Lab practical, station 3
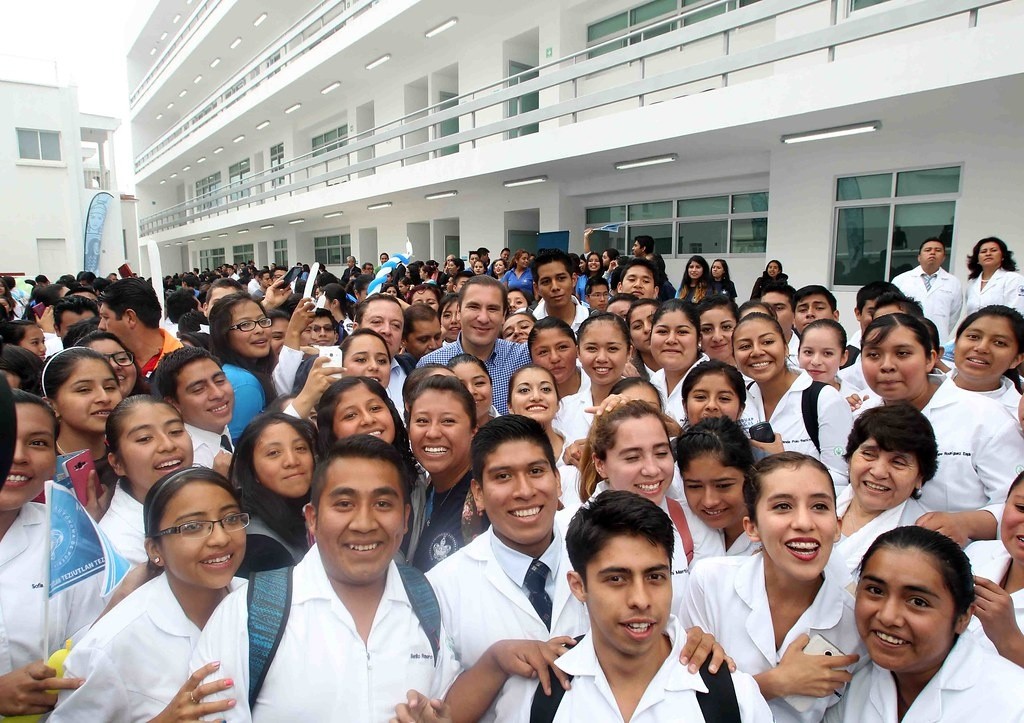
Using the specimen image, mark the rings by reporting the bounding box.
[191,692,197,703]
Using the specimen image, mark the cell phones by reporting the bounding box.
[276,266,303,289]
[319,346,343,379]
[32,302,45,319]
[749,422,775,443]
[63,449,103,506]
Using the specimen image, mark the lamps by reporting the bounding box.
[324,212,343,218]
[164,229,249,247]
[368,54,391,71]
[149,0,301,184]
[426,191,459,199]
[781,119,882,144]
[427,17,458,37]
[321,81,341,95]
[289,219,304,224]
[368,202,393,209]
[261,224,275,229]
[614,153,678,170]
[503,174,547,188]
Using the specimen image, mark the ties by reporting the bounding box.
[922,273,937,291]
[220,434,232,453]
[523,560,553,634]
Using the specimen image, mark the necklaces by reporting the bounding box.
[56,441,65,454]
[982,280,989,282]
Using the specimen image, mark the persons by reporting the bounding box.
[0,228,1024,723]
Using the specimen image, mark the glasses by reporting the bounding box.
[229,318,272,332]
[103,351,135,366]
[314,323,337,333]
[151,511,252,539]
